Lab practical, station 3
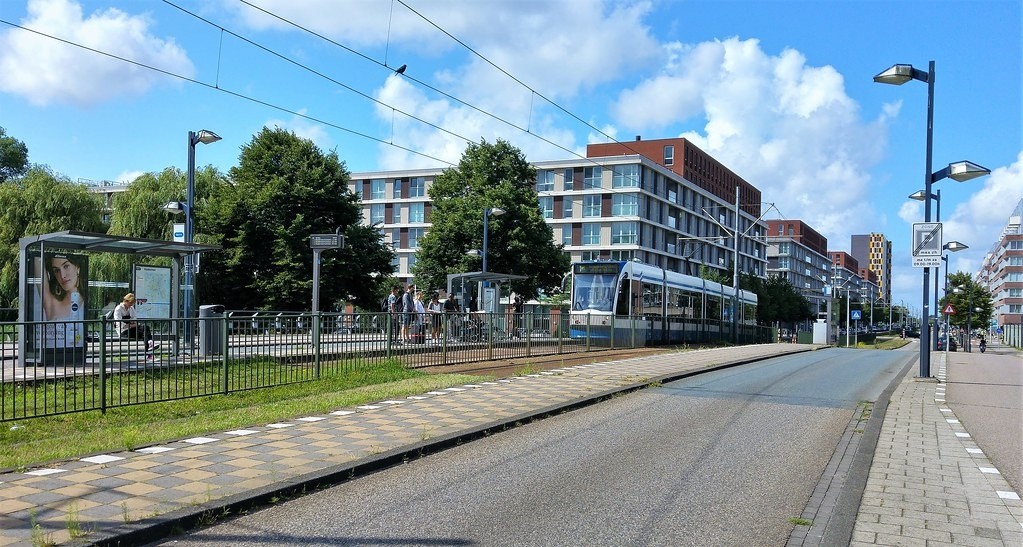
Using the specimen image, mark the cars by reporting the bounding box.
[838,324,921,339]
[938,331,957,351]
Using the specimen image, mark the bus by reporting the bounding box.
[561,242,757,347]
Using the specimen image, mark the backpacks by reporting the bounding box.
[396,292,409,312]
[381,294,389,311]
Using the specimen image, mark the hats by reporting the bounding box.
[124,293,134,303]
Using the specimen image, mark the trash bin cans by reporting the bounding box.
[199,305,224,355]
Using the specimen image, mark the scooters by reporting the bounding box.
[979,341,989,353]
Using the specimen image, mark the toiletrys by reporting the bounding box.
[69,293,79,330]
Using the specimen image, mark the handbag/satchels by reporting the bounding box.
[100,310,114,325]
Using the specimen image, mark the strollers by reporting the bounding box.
[453,315,487,343]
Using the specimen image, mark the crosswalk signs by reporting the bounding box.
[851,310,861,320]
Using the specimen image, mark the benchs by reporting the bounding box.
[87,334,176,355]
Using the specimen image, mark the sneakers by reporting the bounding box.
[148,342,159,350]
[146,354,160,362]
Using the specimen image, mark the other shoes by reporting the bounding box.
[401,339,414,345]
[391,340,403,346]
[433,338,440,343]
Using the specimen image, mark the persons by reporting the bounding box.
[414,291,429,345]
[427,293,443,344]
[979,337,986,346]
[453,299,463,337]
[469,294,478,320]
[512,297,523,326]
[442,293,458,344]
[388,285,403,346]
[402,284,418,344]
[34,256,84,347]
[113,293,161,362]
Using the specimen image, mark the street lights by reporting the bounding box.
[908,189,971,351]
[465,207,507,272]
[163,129,223,343]
[940,254,964,351]
[872,60,993,378]
